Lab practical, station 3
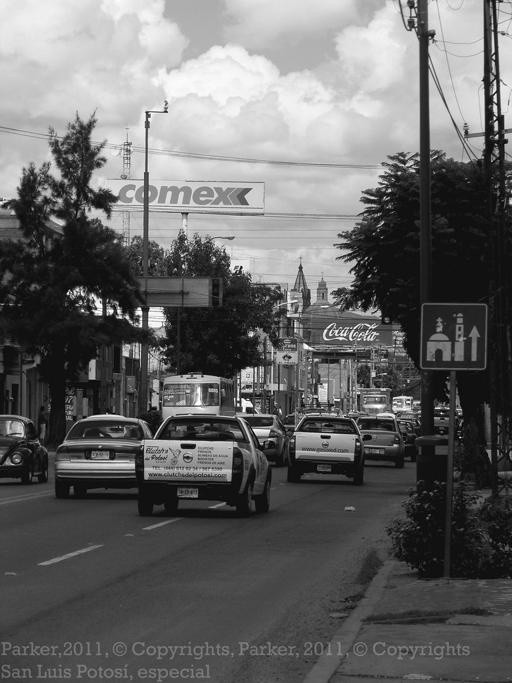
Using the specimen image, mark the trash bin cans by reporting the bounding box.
[415,434,454,485]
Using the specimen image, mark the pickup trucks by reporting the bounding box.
[285,412,372,485]
[135,412,272,517]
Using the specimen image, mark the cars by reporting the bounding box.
[54,411,159,500]
[282,387,464,462]
[355,415,406,468]
[231,412,291,466]
[1,414,49,484]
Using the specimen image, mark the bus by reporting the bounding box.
[159,371,235,425]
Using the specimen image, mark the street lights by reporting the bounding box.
[293,304,330,386]
[369,372,387,387]
[253,298,298,413]
[177,235,235,376]
